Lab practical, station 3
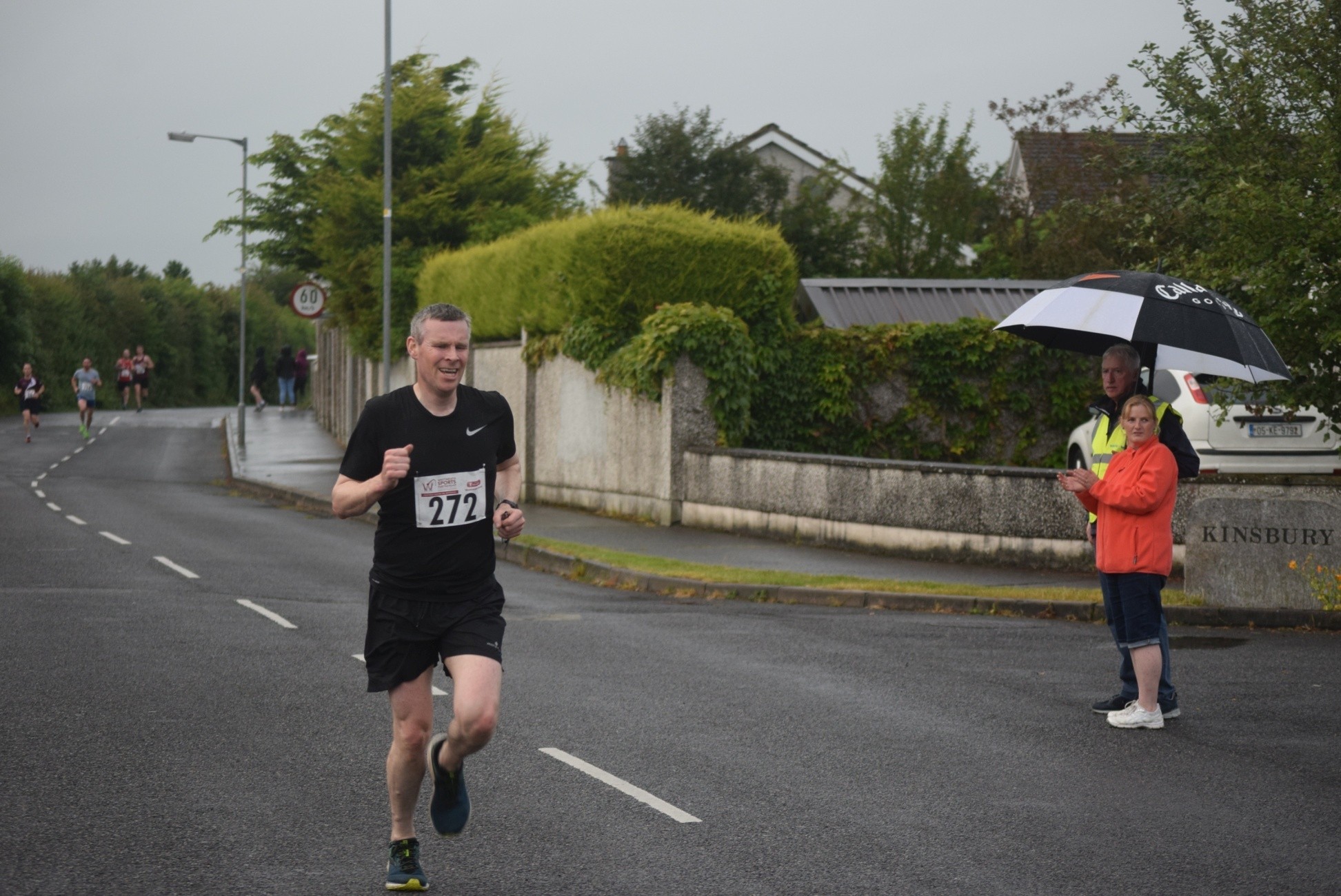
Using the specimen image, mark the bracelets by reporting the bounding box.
[494,499,518,510]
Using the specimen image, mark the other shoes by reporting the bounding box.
[79,423,89,440]
[24,434,31,442]
[254,400,267,413]
[34,422,39,429]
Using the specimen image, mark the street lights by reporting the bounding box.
[168,130,248,451]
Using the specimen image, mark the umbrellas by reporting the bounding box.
[993,267,1291,388]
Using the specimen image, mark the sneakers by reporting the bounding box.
[385,837,430,891]
[1092,694,1140,714]
[1157,692,1181,719]
[1106,700,1165,730]
[427,732,472,839]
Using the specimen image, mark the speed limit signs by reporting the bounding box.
[291,283,326,319]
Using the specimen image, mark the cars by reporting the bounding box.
[1141,364,1341,476]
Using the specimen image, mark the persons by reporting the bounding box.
[13,364,45,441]
[132,346,153,412]
[72,359,102,438]
[115,349,134,409]
[1084,342,1200,718]
[276,344,295,406]
[331,301,526,887]
[1056,395,1178,731]
[292,349,310,405]
[249,347,268,411]
